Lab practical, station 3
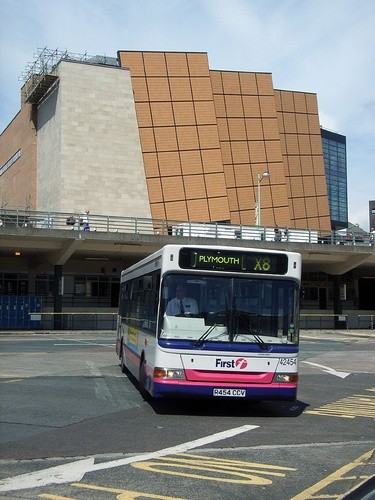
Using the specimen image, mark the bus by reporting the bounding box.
[115,244,305,407]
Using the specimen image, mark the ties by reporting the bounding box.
[179,300,185,315]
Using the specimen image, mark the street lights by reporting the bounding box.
[256,172,270,227]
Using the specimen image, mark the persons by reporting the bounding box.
[163,285,198,317]
[82,211,89,231]
